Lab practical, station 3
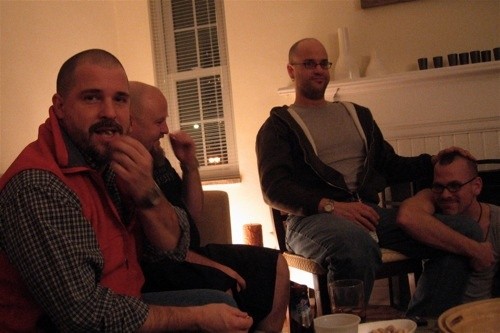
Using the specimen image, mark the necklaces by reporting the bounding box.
[477,201,483,223]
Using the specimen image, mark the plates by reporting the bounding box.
[437,298,500,333]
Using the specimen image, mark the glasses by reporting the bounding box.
[431,178,474,193]
[292,62,332,69]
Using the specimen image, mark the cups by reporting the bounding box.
[481,50,492,62]
[470,51,480,64]
[448,54,458,66]
[328,279,366,322]
[493,47,500,61]
[433,56,443,68]
[459,53,469,65]
[314,313,361,333]
[418,58,427,70]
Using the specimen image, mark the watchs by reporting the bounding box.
[323,199,335,213]
[136,181,163,209]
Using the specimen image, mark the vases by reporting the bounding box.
[334,27,360,81]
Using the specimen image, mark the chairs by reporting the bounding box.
[272,207,423,317]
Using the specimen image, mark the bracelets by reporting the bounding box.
[179,159,199,172]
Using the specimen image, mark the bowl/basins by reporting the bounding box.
[358,318,417,333]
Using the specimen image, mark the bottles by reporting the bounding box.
[352,194,379,243]
[334,28,359,80]
[288,284,316,333]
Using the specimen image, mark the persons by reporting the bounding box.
[255,38,500,333]
[0,49,291,333]
